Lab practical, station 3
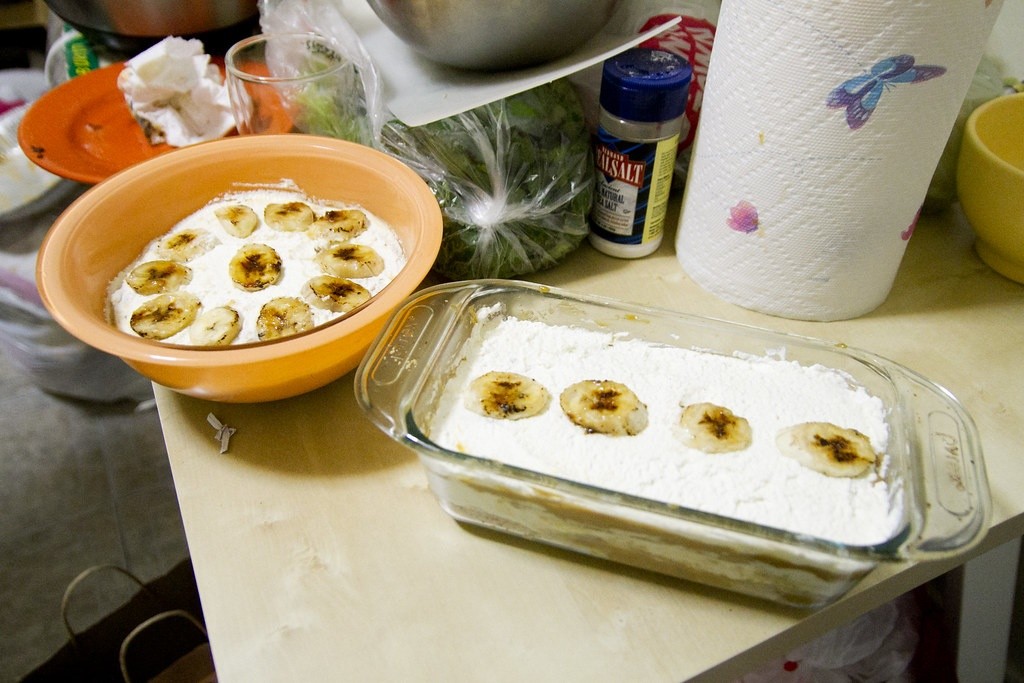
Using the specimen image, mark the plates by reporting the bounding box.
[16,59,296,184]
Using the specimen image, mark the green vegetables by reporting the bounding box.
[292,32,596,280]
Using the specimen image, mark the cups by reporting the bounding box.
[226,32,364,143]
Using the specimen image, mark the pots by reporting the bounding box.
[44,0,271,63]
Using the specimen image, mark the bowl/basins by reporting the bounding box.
[352,278,995,617]
[0,102,83,225]
[369,1,624,76]
[34,135,443,405]
[954,91,1024,289]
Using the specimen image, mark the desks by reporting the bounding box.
[150,198,1023,683]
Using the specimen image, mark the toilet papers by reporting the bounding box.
[670,1,1006,328]
